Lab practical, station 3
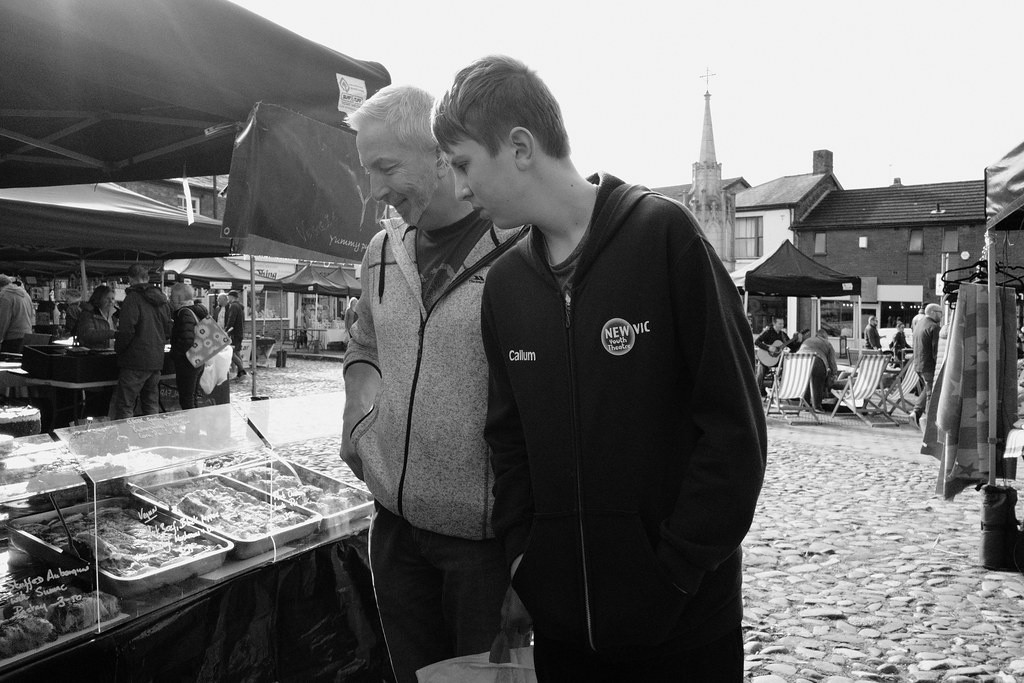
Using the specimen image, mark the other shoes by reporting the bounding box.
[815,409,825,414]
[909,412,920,430]
[235,370,246,382]
[763,395,767,402]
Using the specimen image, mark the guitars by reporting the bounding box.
[757,328,810,367]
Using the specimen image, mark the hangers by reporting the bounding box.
[941,255,1024,309]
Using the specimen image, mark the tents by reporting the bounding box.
[0,1,391,401]
[0,183,362,350]
[729,237,862,359]
[983,142,1024,573]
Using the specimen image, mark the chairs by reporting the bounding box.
[871,355,920,418]
[829,350,900,429]
[766,352,820,427]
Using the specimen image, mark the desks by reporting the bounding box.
[0,513,397,683]
[281,328,327,351]
[306,327,346,350]
[242,337,276,368]
[5,370,178,428]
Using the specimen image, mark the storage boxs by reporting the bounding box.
[20,345,177,384]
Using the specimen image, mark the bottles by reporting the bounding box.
[53,305,60,324]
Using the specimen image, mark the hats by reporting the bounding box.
[228,290,239,298]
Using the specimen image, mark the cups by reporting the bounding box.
[109,338,116,349]
[43,286,50,301]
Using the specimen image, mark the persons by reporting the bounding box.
[340,55,767,683]
[168,282,205,410]
[212,290,247,381]
[795,330,838,414]
[345,297,357,339]
[78,285,120,349]
[296,303,310,348]
[865,316,887,350]
[0,274,32,353]
[908,301,942,430]
[889,321,911,361]
[64,288,81,337]
[754,314,802,402]
[114,263,171,420]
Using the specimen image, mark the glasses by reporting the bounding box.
[934,310,943,314]
[874,320,878,322]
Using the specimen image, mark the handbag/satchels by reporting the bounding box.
[177,308,232,369]
[415,625,539,683]
